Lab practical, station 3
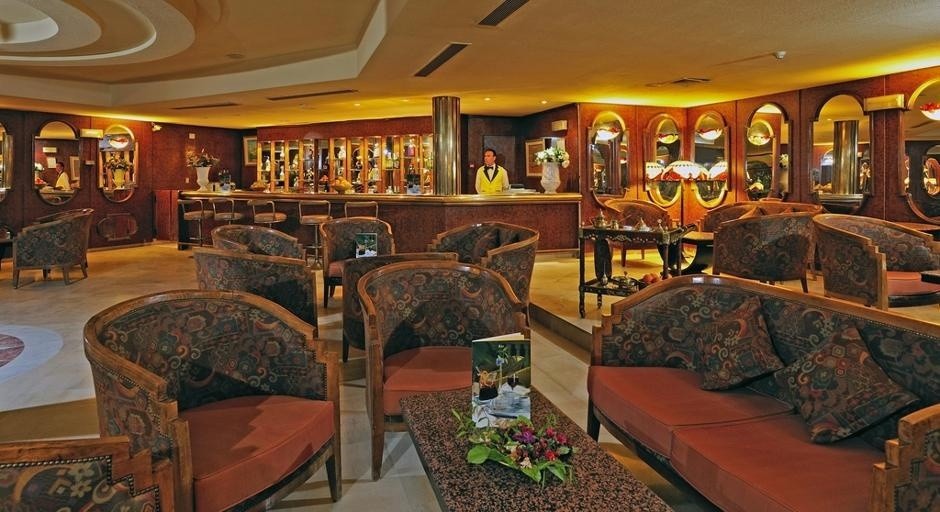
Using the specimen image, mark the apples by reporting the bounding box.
[642,272,672,284]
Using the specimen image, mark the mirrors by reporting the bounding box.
[96,122,140,204]
[33,134,80,196]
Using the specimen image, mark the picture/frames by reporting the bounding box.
[525,139,546,178]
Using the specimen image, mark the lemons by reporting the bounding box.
[332,176,351,186]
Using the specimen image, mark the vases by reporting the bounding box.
[111,168,126,189]
[196,166,211,191]
[539,162,561,196]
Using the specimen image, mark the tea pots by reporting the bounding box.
[590,208,609,228]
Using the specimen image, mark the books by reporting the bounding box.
[469,331,533,429]
[354,232,380,258]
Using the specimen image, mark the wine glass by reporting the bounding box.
[476,378,500,415]
[507,369,519,397]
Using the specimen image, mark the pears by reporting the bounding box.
[250,181,263,189]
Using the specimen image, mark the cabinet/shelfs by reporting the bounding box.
[254,132,432,194]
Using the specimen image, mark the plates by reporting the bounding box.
[512,406,530,413]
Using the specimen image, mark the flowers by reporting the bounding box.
[183,148,220,169]
[534,145,570,169]
[103,153,134,172]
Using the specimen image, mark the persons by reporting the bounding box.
[474,148,510,195]
[53,161,72,190]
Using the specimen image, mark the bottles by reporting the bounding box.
[653,219,665,232]
[264,154,272,169]
[279,164,285,179]
[338,146,346,159]
[385,152,400,169]
[386,184,393,193]
[322,180,331,193]
[406,138,414,156]
[279,144,285,160]
[322,157,330,170]
[220,167,232,194]
[612,219,619,229]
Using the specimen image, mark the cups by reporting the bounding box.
[516,395,530,408]
[488,399,509,414]
[358,242,373,256]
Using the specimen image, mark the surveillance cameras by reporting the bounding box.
[154,125,163,130]
